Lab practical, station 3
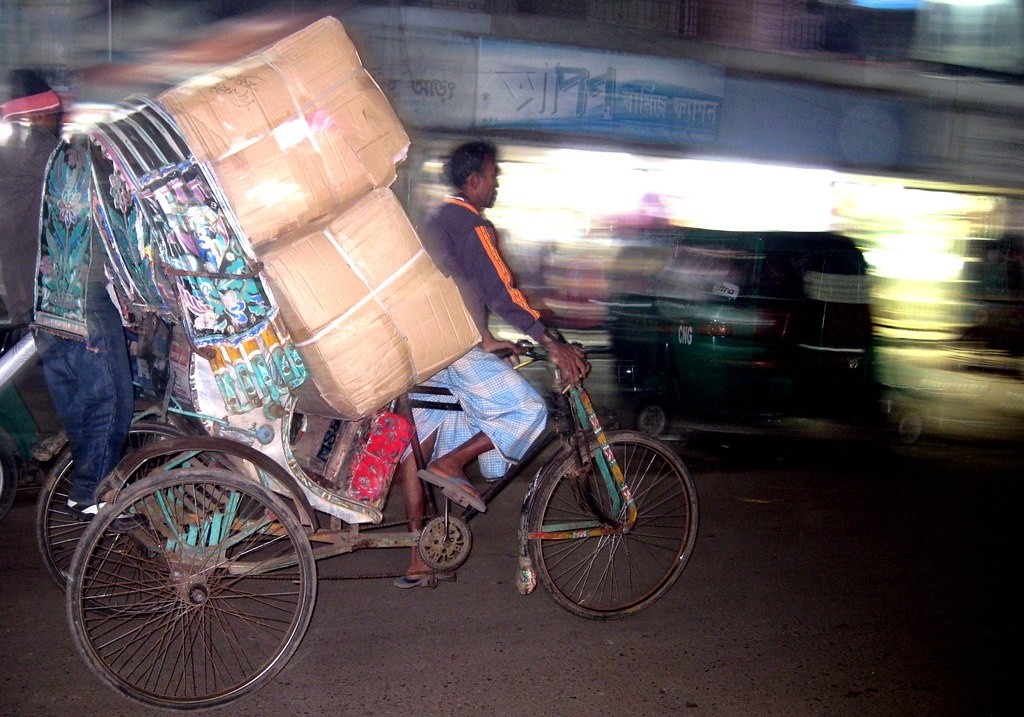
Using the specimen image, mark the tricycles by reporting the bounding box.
[32,308,702,713]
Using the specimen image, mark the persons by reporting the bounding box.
[0,68,142,535]
[389,139,590,587]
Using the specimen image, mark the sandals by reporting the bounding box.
[65,496,149,535]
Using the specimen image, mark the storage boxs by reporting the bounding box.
[77,8,410,246]
[254,185,482,423]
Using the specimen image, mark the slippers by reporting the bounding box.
[393,570,457,588]
[416,468,487,512]
